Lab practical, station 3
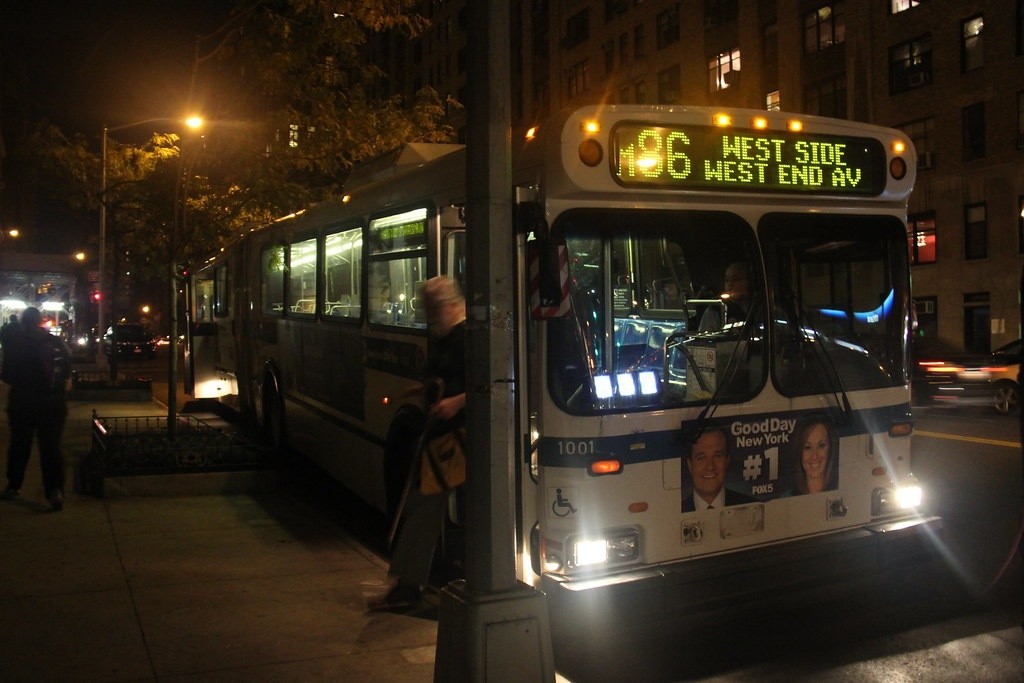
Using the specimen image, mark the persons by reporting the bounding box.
[697,260,794,346]
[366,273,482,613]
[684,416,839,514]
[0,306,71,510]
[0,313,74,373]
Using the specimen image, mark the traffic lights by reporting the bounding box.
[182,263,190,277]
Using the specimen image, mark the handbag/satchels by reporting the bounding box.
[381,402,431,490]
[41,333,81,403]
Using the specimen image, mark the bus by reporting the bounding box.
[188,101,945,623]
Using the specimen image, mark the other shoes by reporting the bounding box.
[46,490,66,509]
[0,486,22,500]
[367,584,418,608]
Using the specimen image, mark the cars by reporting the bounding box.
[88,318,157,363]
[980,339,1024,416]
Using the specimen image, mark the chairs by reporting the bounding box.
[612,318,689,370]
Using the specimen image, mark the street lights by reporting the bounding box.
[94,111,208,373]
